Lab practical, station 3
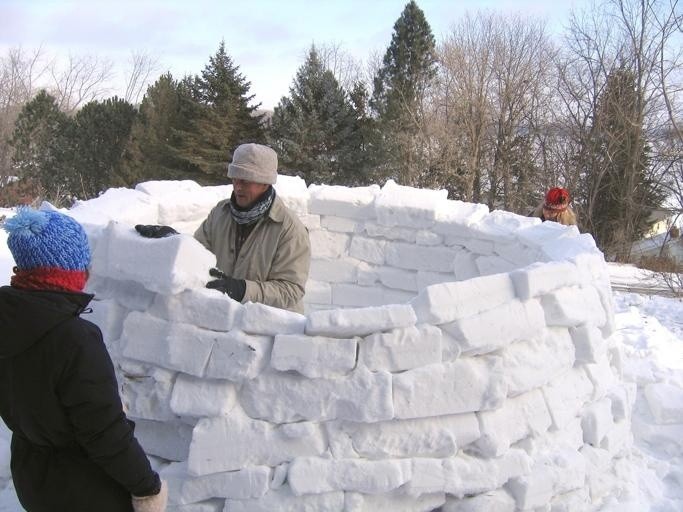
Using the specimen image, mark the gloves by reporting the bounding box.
[134,224,178,238]
[207,264,245,301]
[131,474,169,512]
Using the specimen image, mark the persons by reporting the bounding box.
[526,186,576,229]
[0,203,170,511]
[133,141,312,313]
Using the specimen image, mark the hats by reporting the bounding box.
[226,142,278,187]
[543,187,570,212]
[2,205,92,274]
